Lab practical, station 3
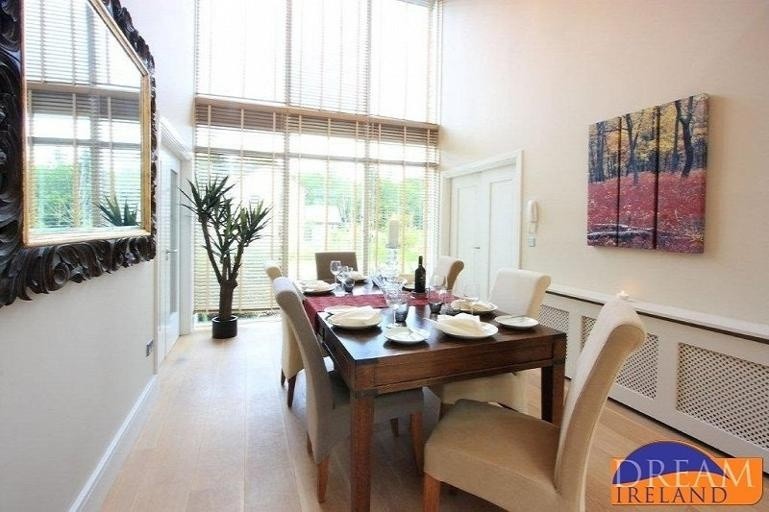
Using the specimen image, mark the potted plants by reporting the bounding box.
[176,172,276,340]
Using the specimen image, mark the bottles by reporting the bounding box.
[414,256,427,294]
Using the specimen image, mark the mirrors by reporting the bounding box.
[1,1,159,309]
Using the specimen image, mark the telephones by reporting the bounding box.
[528,200,537,222]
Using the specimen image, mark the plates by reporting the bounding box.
[324,298,539,346]
[299,277,336,296]
[403,282,431,290]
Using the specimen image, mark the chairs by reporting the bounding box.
[263,249,646,512]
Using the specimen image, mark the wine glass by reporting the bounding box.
[328,259,356,294]
[363,267,411,329]
[427,272,476,315]
